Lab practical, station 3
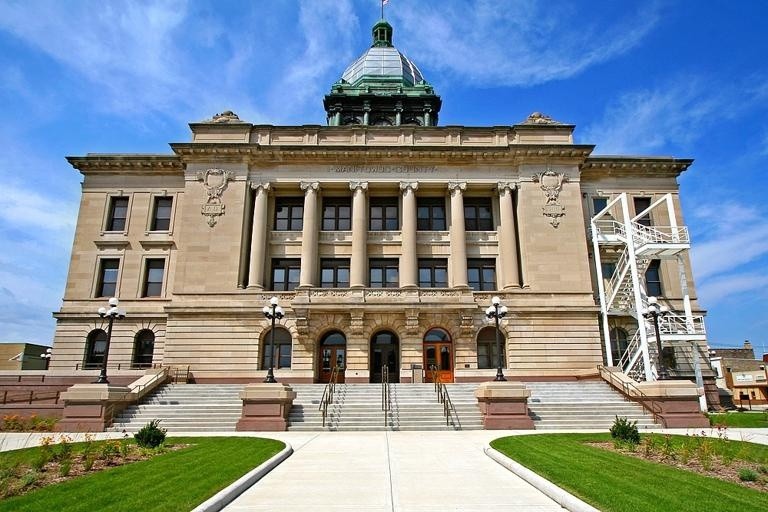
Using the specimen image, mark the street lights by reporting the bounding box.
[261,296,283,383]
[89,297,128,385]
[38,349,51,370]
[483,295,508,381]
